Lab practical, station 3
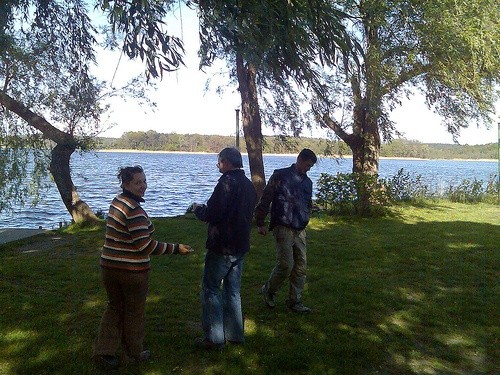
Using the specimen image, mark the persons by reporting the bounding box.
[193,146,257,351]
[94,164,195,375]
[255,148,317,312]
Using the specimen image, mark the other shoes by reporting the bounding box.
[197,337,244,350]
[129,343,156,364]
[285,298,310,311]
[261,285,276,308]
[96,354,122,371]
